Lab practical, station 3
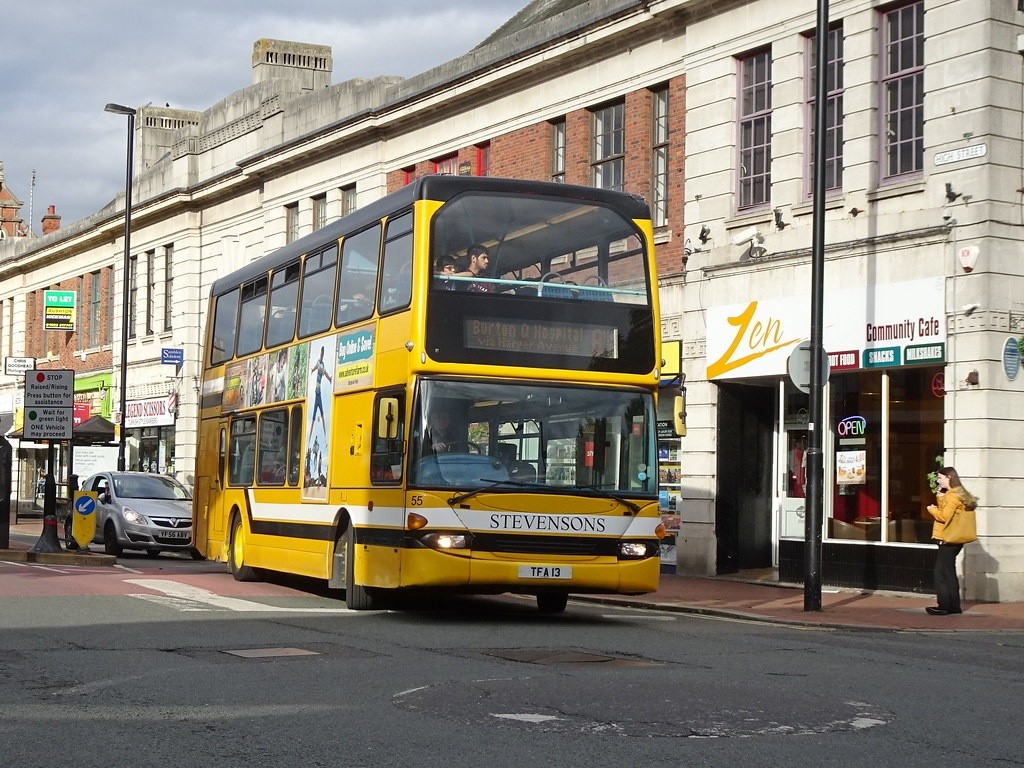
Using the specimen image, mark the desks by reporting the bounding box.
[854,520,880,530]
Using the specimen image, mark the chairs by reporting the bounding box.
[223,295,333,360]
[828,515,938,544]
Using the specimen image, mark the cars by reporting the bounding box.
[63,471,208,562]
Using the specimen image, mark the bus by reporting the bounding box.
[186,173,690,612]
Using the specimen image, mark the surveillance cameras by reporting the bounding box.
[732,227,758,246]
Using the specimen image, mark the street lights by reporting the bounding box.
[104,103,137,471]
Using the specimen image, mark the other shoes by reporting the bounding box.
[926,607,962,614]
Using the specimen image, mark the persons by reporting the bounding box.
[926,467,978,615]
[98,482,112,502]
[338,276,371,324]
[434,244,517,296]
[421,408,468,457]
[249,346,332,485]
